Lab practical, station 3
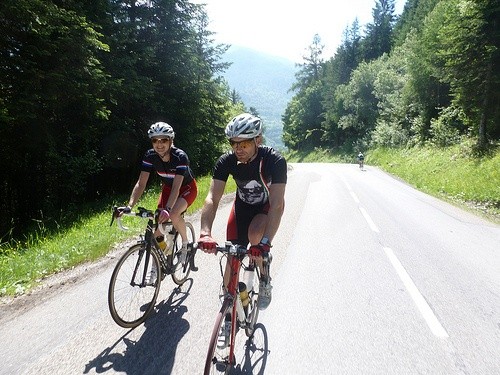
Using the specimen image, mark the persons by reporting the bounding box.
[198,113,288,349]
[357,152,364,167]
[116,122,198,284]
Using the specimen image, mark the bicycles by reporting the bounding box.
[359,159,363,169]
[195,240,272,373]
[108,206,198,328]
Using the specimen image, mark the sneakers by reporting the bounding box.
[256,283,272,310]
[217,326,231,349]
[145,270,157,284]
[181,245,188,264]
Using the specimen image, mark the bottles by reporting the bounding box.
[165,232,174,256]
[243,264,255,299]
[156,236,167,252]
[239,282,249,308]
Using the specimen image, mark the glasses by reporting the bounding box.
[230,138,255,149]
[150,138,169,144]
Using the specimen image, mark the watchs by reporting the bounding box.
[260,238,271,247]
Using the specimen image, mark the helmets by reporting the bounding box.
[225,113,262,138]
[148,121,174,139]
